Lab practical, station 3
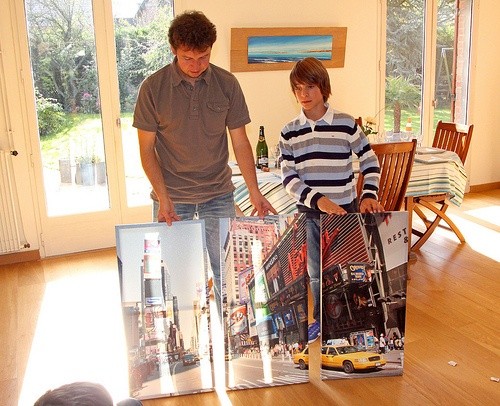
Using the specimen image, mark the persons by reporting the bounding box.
[244,332,399,354]
[34,381,143,406]
[281,57,386,345]
[132,10,281,323]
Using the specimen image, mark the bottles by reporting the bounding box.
[256,126,268,168]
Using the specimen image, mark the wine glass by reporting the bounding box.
[271,144,281,171]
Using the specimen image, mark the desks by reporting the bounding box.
[228,146,469,261]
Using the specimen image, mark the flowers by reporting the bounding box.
[361,106,388,137]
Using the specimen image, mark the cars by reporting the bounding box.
[293,347,309,370]
[183,354,196,366]
[321,344,386,374]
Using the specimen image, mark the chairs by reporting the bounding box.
[356,139,417,212]
[410,120,474,253]
